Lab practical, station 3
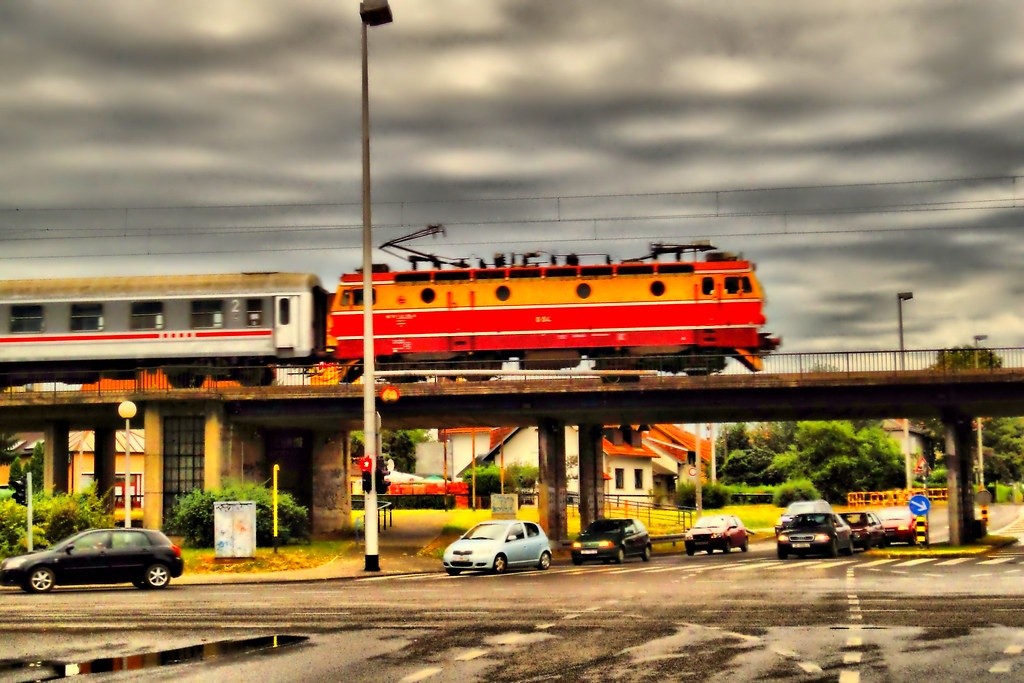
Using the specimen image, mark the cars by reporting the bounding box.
[0,527,185,594]
[837,512,887,550]
[441,519,554,576]
[774,512,855,559]
[570,518,652,565]
[877,505,917,547]
[684,514,749,557]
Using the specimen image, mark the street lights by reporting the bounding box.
[897,291,914,490]
[118,400,137,544]
[974,334,988,533]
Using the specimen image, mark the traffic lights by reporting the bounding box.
[359,457,373,494]
[375,454,392,494]
[9,478,27,503]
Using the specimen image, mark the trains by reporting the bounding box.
[0,240,784,389]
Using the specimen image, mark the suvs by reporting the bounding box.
[774,499,835,538]
